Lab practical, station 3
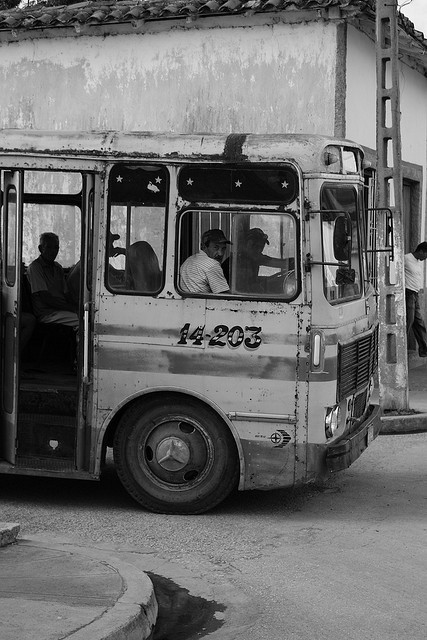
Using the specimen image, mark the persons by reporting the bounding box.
[180,229,231,293]
[404,241,426,359]
[220,228,294,293]
[66,231,126,303]
[27,232,79,372]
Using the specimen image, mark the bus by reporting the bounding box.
[0,128,382,514]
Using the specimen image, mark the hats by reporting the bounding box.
[108,230,119,240]
[247,228,268,245]
[201,229,233,246]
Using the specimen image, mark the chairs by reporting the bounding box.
[126,242,160,291]
